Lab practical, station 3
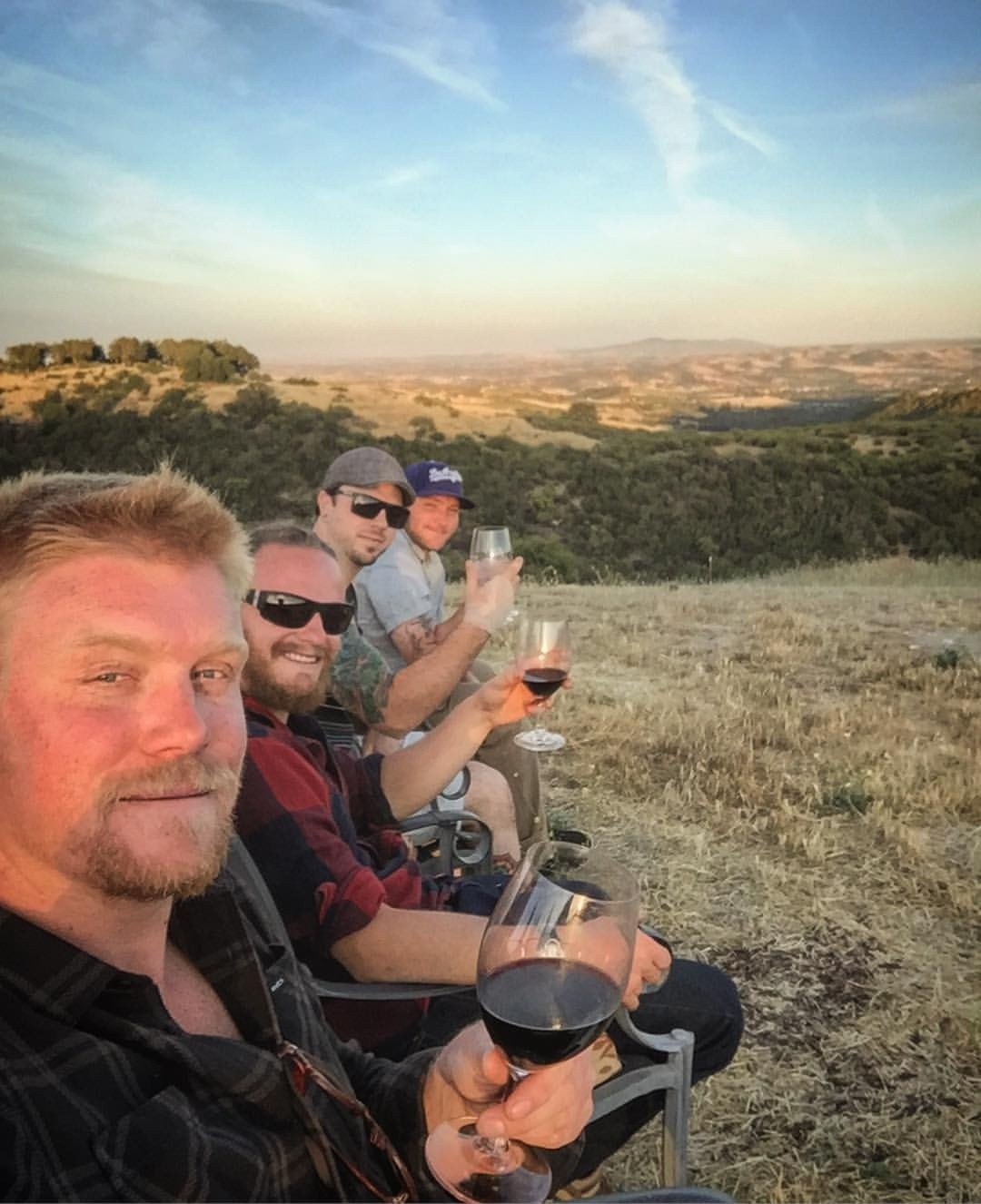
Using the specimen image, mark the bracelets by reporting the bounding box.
[537,923,567,963]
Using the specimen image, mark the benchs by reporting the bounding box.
[221,691,691,1187]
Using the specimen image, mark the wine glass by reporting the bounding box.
[513,618,571,752]
[471,525,521,630]
[422,841,638,1204]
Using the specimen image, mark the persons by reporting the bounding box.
[0,460,737,1204]
[311,446,521,867]
[233,520,743,1202]
[352,461,594,871]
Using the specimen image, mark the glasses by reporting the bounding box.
[334,490,410,528]
[242,589,355,635]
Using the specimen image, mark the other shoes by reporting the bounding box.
[543,827,594,872]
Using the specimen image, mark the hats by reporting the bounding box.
[322,446,416,506]
[405,462,475,509]
